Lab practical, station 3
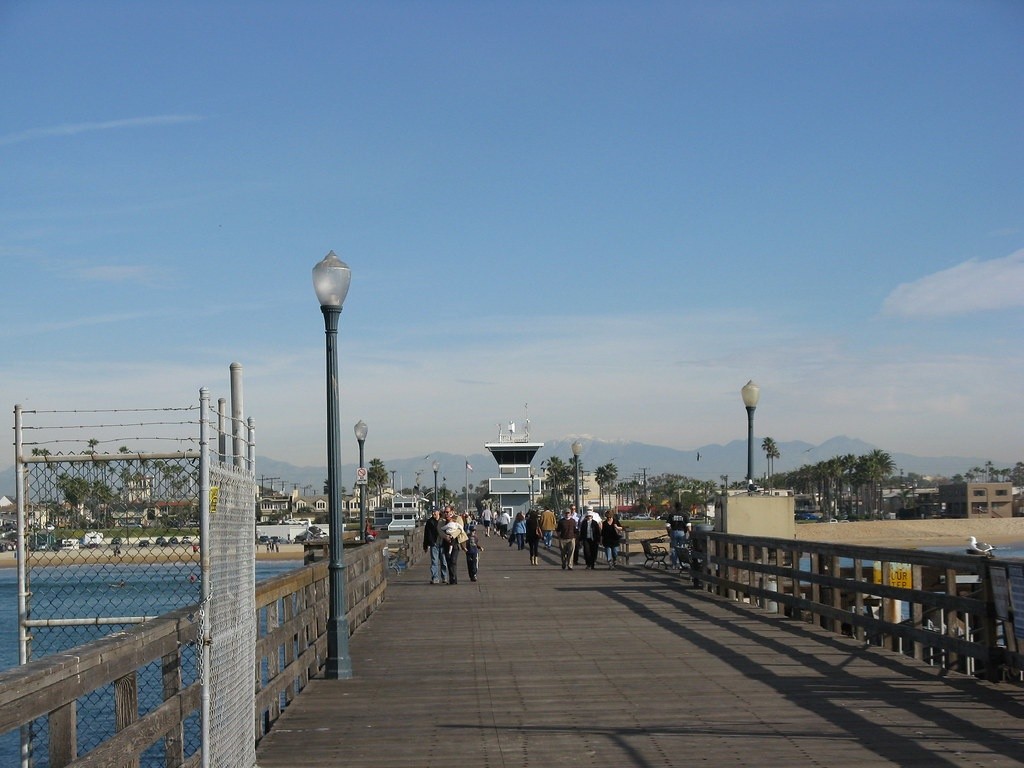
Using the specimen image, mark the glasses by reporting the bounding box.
[444,510,452,513]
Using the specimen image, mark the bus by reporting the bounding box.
[157,515,178,523]
[112,517,151,527]
[30,534,54,550]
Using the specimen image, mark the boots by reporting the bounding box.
[535,556,538,565]
[614,559,617,565]
[609,562,614,569]
[531,556,535,564]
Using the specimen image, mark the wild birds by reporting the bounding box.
[747,479,758,493]
[965,536,995,554]
[696,452,702,462]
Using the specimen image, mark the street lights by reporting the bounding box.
[433,460,440,510]
[390,470,397,491]
[742,379,760,496]
[311,246,354,680]
[616,467,652,506]
[571,441,583,519]
[353,420,369,540]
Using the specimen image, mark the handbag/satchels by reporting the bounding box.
[536,519,543,539]
[614,517,624,537]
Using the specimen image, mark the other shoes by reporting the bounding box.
[471,576,477,581]
[562,564,566,569]
[568,565,573,569]
[450,580,457,583]
[432,580,438,583]
[443,580,448,584]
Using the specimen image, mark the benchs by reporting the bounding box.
[640,540,669,570]
[673,546,693,577]
[389,547,402,575]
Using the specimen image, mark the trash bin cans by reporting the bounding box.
[692,524,714,587]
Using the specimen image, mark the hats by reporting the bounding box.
[469,526,477,530]
[585,511,593,517]
[564,508,570,514]
[433,508,440,511]
[586,506,593,510]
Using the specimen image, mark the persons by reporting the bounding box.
[437,506,464,585]
[557,508,577,570]
[526,512,541,565]
[665,502,691,570]
[541,506,556,552]
[513,513,527,550]
[191,573,195,583]
[497,511,510,540]
[193,543,198,554]
[423,506,448,584]
[578,511,600,570]
[464,526,484,582]
[601,510,622,569]
[441,514,468,554]
[111,546,121,558]
[423,505,623,585]
[119,578,125,589]
[266,540,281,553]
[481,506,493,538]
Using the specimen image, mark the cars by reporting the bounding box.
[830,519,849,523]
[796,513,820,520]
[111,523,192,548]
[259,535,283,543]
[689,515,699,520]
[631,514,655,520]
[285,519,309,525]
[0,518,55,550]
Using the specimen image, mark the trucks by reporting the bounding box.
[84,532,103,549]
[62,539,80,550]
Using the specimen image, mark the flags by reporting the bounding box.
[466,460,474,472]
